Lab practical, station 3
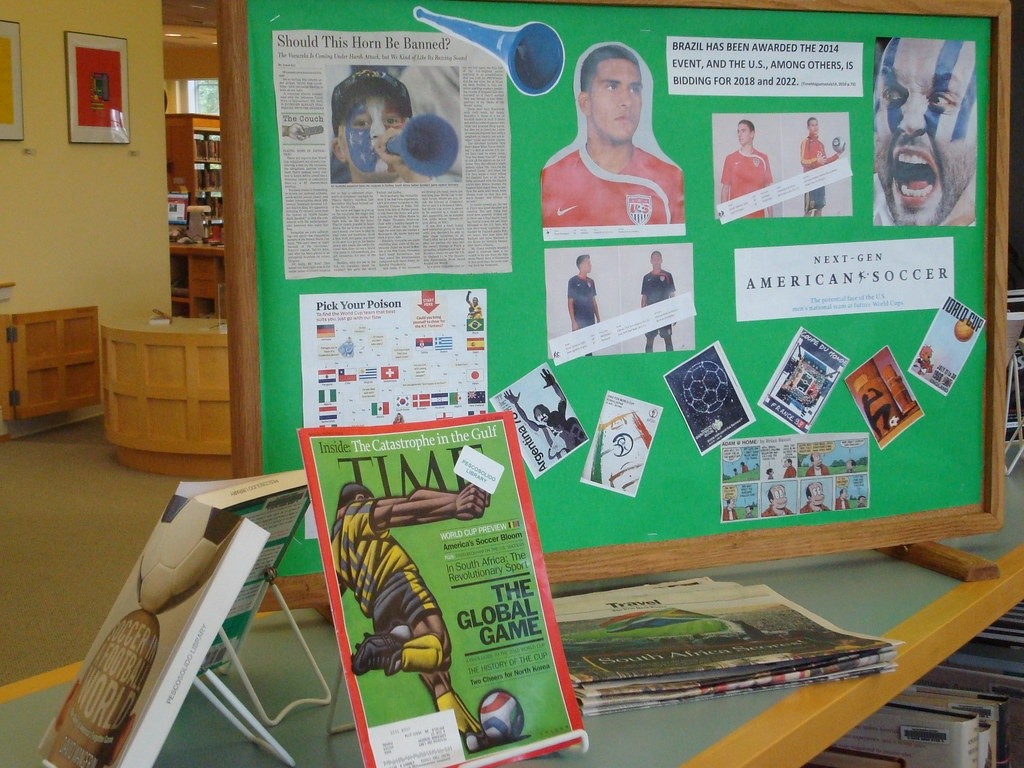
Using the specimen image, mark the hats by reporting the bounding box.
[332,70,413,135]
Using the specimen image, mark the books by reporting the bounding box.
[42,469,311,768]
[800,597,1024,768]
[194,134,222,219]
[298,411,585,767]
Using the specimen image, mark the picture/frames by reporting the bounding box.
[64,30,131,145]
[0,19,25,142]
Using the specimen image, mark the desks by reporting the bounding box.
[100,315,232,479]
[170,242,225,319]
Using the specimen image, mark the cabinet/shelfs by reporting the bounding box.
[0,440,1024,768]
[165,113,223,225]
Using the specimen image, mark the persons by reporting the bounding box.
[874,36,977,227]
[329,69,430,183]
[569,255,600,356]
[641,252,676,352]
[542,46,684,228]
[721,120,773,217]
[802,118,842,217]
